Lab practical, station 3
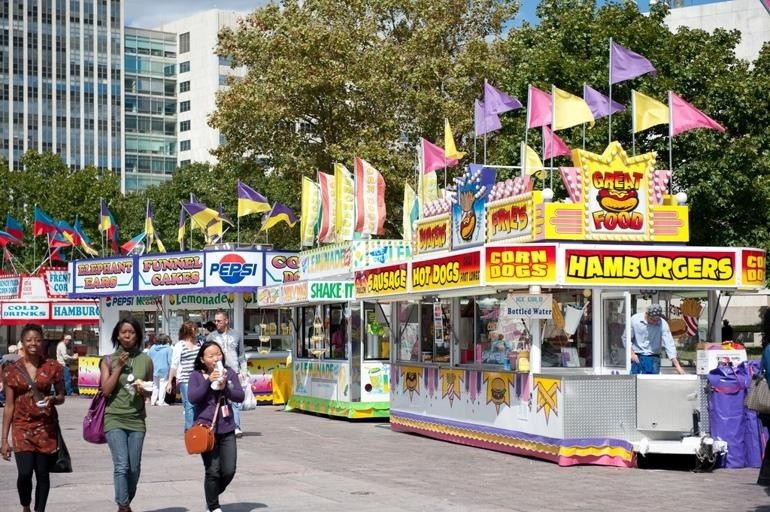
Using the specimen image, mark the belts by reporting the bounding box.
[635,353,660,356]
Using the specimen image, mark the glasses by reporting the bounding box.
[213,318,226,324]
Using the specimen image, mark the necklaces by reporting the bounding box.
[117,348,136,371]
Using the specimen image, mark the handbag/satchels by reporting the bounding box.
[49,419,72,473]
[185,424,214,454]
[83,391,108,443]
[744,374,770,415]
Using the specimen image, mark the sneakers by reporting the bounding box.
[235,429,243,437]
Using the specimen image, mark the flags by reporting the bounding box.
[681,311,698,337]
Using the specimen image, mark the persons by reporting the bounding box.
[757,307,769,487]
[1,323,64,512]
[0,341,25,407]
[142,334,175,406]
[165,320,201,431]
[56,334,79,396]
[195,320,217,343]
[332,325,344,358]
[206,311,245,437]
[623,304,686,375]
[189,341,245,511]
[722,319,733,340]
[99,316,154,511]
